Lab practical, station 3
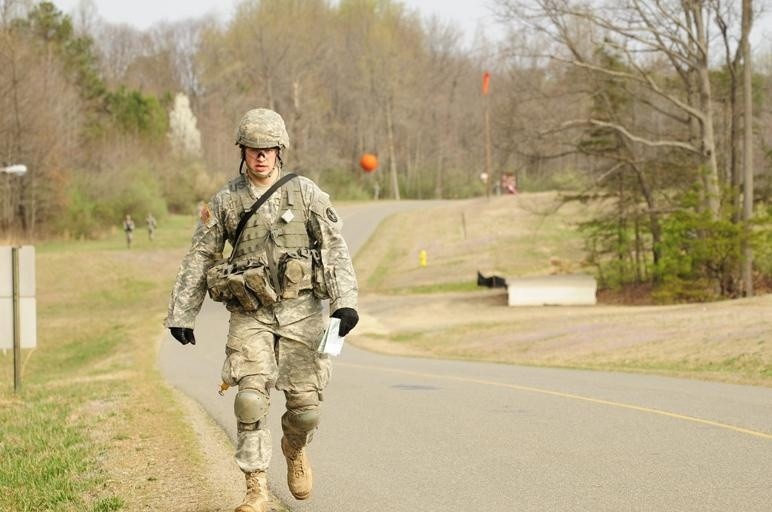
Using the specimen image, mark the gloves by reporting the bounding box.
[170,327,195,345]
[332,307,358,336]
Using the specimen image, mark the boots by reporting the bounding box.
[282,435,312,500]
[235,472,269,512]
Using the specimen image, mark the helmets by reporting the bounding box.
[235,109,290,150]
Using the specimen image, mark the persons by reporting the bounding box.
[123,215,134,248]
[162,107,359,512]
[146,212,157,241]
